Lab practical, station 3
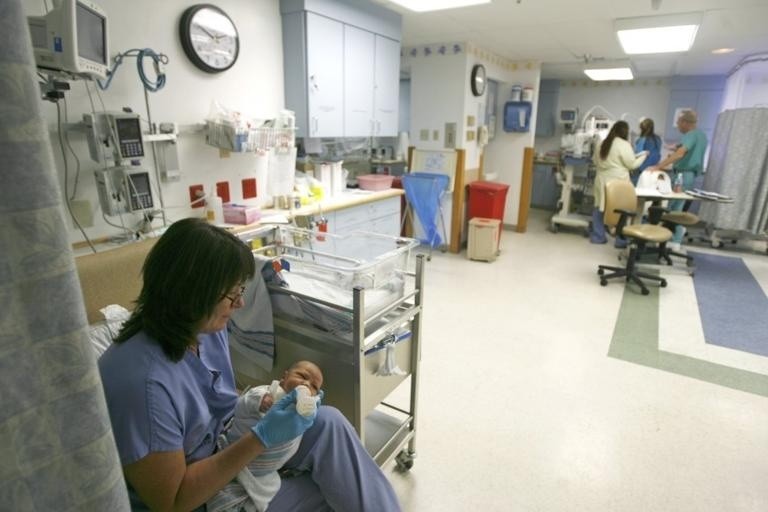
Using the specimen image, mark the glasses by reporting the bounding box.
[221,286,247,305]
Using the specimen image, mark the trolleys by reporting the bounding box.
[236,224,424,473]
[549,106,768,255]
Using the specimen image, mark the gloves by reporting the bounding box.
[251,391,326,447]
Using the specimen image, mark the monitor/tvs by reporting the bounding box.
[27,1,111,78]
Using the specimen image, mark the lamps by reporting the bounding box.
[614,12,703,56]
[582,59,637,82]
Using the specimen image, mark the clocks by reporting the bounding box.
[179,4,239,73]
[471,65,486,96]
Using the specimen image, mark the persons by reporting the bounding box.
[258,359,324,418]
[647,108,709,251]
[589,119,650,248]
[630,117,662,224]
[95,217,404,512]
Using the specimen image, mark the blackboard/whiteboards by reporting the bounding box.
[411,147,458,194]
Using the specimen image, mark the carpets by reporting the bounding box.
[606,249,767,397]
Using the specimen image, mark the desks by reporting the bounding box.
[634,191,736,226]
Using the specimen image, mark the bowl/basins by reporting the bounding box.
[357,174,395,191]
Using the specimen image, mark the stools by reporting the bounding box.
[598,179,673,295]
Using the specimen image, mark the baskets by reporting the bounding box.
[208,120,299,152]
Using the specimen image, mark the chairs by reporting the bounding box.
[74,235,161,324]
[636,167,700,266]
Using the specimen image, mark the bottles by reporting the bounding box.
[205,189,224,226]
[295,384,315,417]
[673,173,683,193]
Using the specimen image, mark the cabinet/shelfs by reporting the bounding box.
[280,1,403,138]
[234,191,406,251]
